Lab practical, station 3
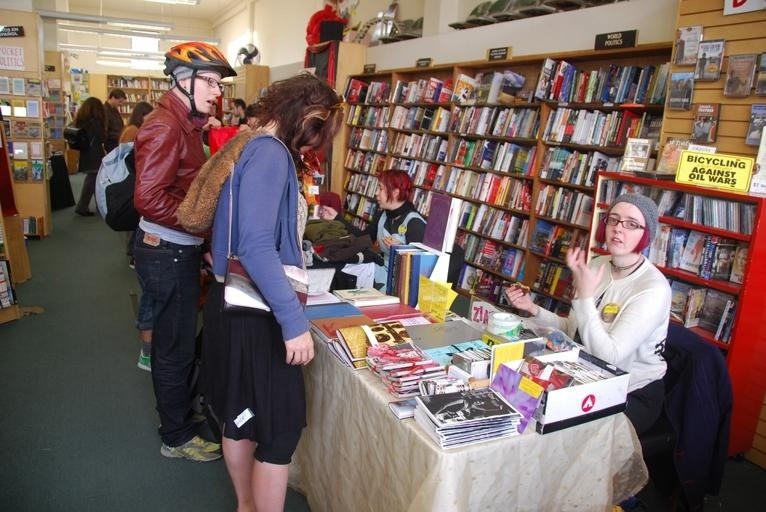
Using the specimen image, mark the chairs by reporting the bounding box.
[608,319,731,511]
[444,241,463,285]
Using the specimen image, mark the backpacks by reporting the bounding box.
[64,118,97,151]
[95,142,141,232]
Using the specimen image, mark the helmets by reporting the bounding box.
[163,42,237,79]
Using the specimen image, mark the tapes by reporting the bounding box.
[486,311,522,338]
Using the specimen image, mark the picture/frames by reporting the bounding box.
[1,75,44,162]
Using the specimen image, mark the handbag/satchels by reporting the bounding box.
[223,254,309,316]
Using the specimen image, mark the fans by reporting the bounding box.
[234,42,263,66]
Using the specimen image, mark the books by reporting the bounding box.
[667,190,749,346]
[0,73,90,183]
[343,77,452,234]
[695,38,726,83]
[750,131,764,194]
[528,58,670,314]
[620,137,653,173]
[672,25,704,67]
[106,76,173,114]
[669,72,695,111]
[746,104,763,146]
[657,137,692,174]
[442,71,542,303]
[304,286,613,450]
[222,86,235,98]
[689,144,716,155]
[724,55,762,97]
[690,103,720,143]
[411,188,434,217]
[599,178,756,237]
[18,214,44,235]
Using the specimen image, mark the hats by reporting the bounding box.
[606,193,657,245]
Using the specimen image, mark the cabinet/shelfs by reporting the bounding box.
[522,42,675,317]
[578,167,766,453]
[389,66,458,221]
[218,82,235,125]
[151,74,171,114]
[447,55,542,314]
[107,73,149,128]
[341,71,397,230]
[232,65,270,109]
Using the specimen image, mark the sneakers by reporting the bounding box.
[161,435,224,462]
[138,350,151,371]
[74,208,95,216]
[158,412,207,435]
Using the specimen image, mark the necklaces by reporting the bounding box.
[609,255,641,272]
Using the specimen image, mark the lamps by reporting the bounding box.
[34,1,220,64]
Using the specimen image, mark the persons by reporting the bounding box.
[673,39,685,64]
[726,70,747,97]
[502,192,674,440]
[319,169,428,274]
[134,273,155,373]
[202,98,268,161]
[131,40,238,464]
[66,91,154,217]
[733,245,748,281]
[195,72,347,512]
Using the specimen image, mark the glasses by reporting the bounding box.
[195,75,224,92]
[605,218,646,229]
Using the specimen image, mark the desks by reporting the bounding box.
[305,314,626,511]
